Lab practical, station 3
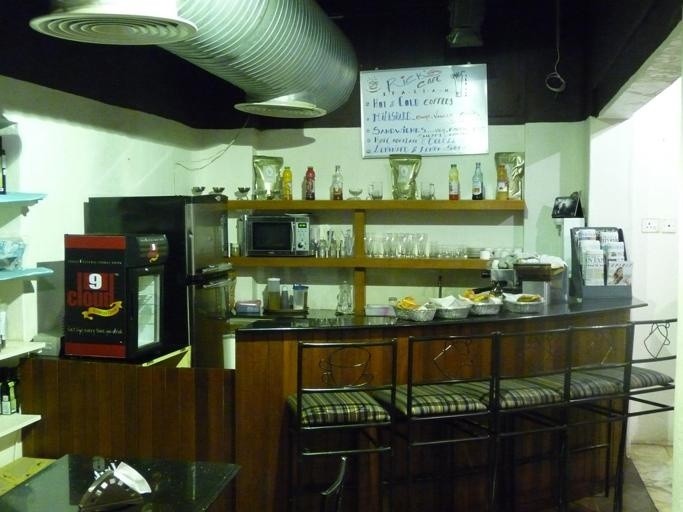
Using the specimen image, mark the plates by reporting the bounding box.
[397,293,546,322]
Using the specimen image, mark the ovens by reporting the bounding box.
[83,191,236,377]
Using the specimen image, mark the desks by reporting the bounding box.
[0,450,241,511]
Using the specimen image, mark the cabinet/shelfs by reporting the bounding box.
[0,192,58,499]
[229,198,524,320]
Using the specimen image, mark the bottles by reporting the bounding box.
[448,164,460,200]
[318,239,345,260]
[331,165,343,201]
[496,163,509,201]
[282,166,293,201]
[326,229,353,257]
[266,278,308,312]
[335,280,352,315]
[1,395,10,415]
[0,136,7,193]
[8,382,17,413]
[0,373,20,415]
[471,162,484,199]
[305,166,316,200]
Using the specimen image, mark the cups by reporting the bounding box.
[363,231,431,260]
[420,181,435,200]
[371,182,384,201]
[466,246,521,261]
[191,185,250,202]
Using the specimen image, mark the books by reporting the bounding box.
[574,228,632,288]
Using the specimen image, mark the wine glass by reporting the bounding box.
[348,187,363,201]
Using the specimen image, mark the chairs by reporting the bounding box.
[288,338,400,508]
[374,330,498,508]
[452,313,676,511]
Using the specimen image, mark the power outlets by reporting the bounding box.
[641,218,659,233]
